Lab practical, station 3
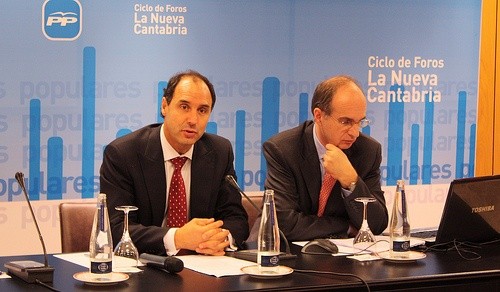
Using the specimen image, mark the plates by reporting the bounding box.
[379,250,427,264]
[73,271,129,286]
[240,265,293,278]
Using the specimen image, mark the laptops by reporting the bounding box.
[382,174,500,247]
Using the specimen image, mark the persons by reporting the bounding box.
[262,76,389,241]
[99,72,250,257]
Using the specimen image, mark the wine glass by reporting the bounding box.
[354,197,378,255]
[114,205,140,270]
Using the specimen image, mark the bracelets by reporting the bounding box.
[345,182,355,190]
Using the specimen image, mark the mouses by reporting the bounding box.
[301,239,338,254]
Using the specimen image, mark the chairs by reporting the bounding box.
[59,201,97,253]
[240,196,264,232]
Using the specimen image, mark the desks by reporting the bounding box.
[0,232,500,292]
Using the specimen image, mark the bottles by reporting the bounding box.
[89,193,114,282]
[391,179,411,259]
[256,189,280,276]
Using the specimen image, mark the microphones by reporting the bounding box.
[3,172,54,284]
[225,175,296,258]
[140,253,185,273]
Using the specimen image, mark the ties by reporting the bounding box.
[317,172,337,217]
[166,157,188,255]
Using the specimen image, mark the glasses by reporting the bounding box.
[327,112,370,128]
